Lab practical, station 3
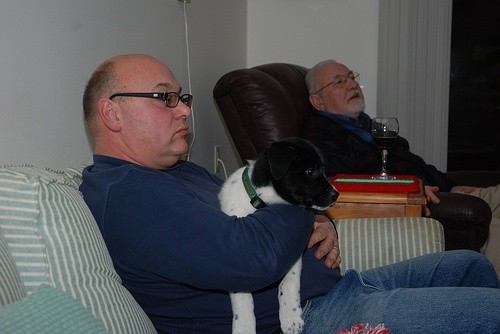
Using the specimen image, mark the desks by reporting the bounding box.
[327,179,426,219]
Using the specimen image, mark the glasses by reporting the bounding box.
[108,92,193,108]
[315,72,361,94]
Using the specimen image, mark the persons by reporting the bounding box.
[77,52,500,333]
[305,58,492,252]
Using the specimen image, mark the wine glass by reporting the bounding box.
[371,116,399,180]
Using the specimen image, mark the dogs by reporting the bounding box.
[219,136,340,334]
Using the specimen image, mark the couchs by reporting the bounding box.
[213,63,492,252]
[0,164,444,334]
